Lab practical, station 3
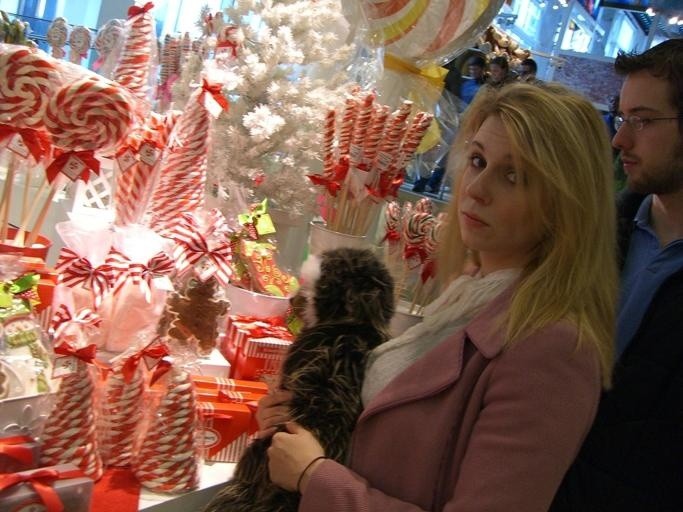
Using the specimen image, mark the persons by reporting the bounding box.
[460,55,485,102]
[487,57,509,81]
[517,58,537,78]
[265,74,622,512]
[549,35,683,511]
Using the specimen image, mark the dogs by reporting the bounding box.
[204,246,396,512]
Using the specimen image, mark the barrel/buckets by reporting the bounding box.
[255,206,315,278]
[308,219,369,259]
[0,222,53,263]
[224,274,301,321]
[388,297,426,340]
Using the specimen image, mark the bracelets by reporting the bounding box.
[295,455,326,495]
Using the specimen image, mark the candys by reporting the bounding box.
[384,198,450,256]
[321,87,438,182]
[339,0,505,70]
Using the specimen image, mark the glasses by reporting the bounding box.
[612,113,681,131]
